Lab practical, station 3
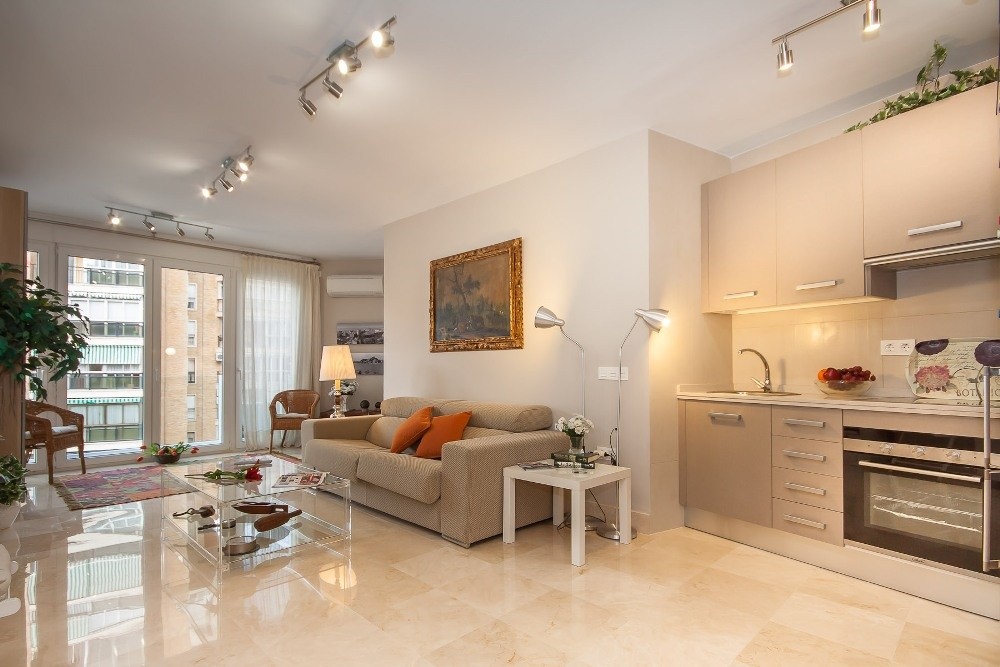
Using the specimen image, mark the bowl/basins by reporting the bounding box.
[814,379,873,397]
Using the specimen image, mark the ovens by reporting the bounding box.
[841,426,1000,583]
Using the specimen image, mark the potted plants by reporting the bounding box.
[1,454,28,530]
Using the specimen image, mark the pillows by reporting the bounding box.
[413,412,473,460]
[390,404,434,455]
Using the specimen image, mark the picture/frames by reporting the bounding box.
[430,236,523,354]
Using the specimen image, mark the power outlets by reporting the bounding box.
[881,340,917,357]
[597,447,617,461]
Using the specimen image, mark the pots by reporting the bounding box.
[198,517,237,531]
[219,535,261,557]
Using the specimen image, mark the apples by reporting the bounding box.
[822,367,841,381]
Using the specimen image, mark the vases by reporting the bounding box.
[339,395,354,413]
[569,436,585,456]
[153,453,182,465]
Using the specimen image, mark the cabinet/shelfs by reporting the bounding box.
[680,399,844,571]
[702,80,1000,316]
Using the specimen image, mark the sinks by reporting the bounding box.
[708,390,802,397]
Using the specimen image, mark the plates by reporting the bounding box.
[906,337,1000,401]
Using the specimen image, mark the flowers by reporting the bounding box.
[328,381,360,398]
[136,442,200,464]
[184,466,264,484]
[555,414,596,450]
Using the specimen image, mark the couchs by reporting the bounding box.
[301,397,573,550]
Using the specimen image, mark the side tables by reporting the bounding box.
[502,459,632,569]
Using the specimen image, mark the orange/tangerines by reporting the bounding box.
[817,369,828,382]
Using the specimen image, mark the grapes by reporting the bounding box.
[831,365,876,390]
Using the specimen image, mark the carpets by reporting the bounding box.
[51,466,199,511]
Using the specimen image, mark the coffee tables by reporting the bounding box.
[162,454,353,584]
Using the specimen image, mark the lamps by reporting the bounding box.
[142,216,156,232]
[372,22,395,49]
[535,306,607,533]
[205,228,215,242]
[108,209,122,228]
[230,162,248,183]
[203,182,219,200]
[320,346,358,421]
[778,38,795,73]
[322,70,344,100]
[300,88,319,118]
[239,149,255,172]
[339,49,362,76]
[863,0,883,34]
[220,173,234,193]
[596,308,671,542]
[176,222,187,238]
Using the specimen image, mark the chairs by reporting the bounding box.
[24,398,87,486]
[270,389,321,456]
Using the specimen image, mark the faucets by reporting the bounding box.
[738,348,772,394]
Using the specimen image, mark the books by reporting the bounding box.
[550,449,600,464]
[271,471,332,488]
[553,460,595,470]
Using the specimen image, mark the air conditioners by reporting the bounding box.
[327,275,385,298]
